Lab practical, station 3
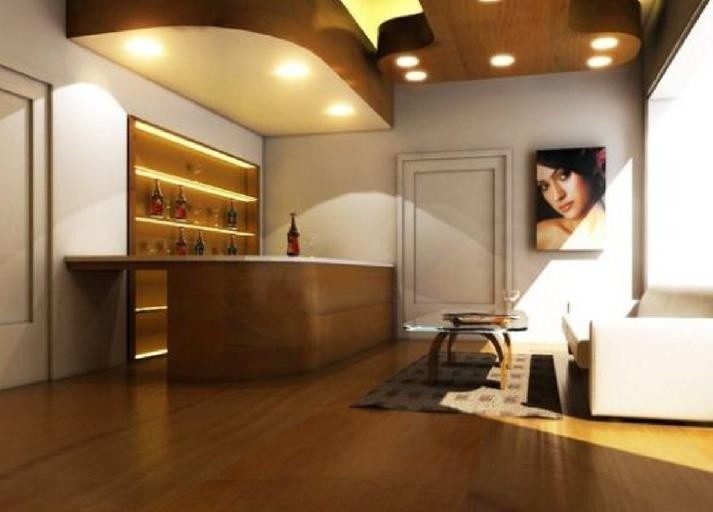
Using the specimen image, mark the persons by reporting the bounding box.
[534,148,606,251]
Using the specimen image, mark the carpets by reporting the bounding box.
[350,348,563,420]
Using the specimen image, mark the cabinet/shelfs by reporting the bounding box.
[128,113,261,365]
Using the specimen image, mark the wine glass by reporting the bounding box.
[502,288,519,318]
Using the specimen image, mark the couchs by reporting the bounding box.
[560,284,713,429]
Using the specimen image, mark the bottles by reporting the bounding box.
[286,213,301,257]
[149,178,238,256]
